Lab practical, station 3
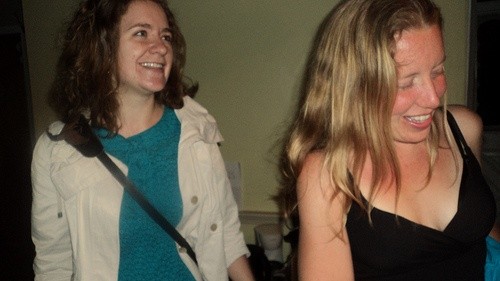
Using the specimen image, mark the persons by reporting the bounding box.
[270,0,500,281]
[28,0,253,281]
